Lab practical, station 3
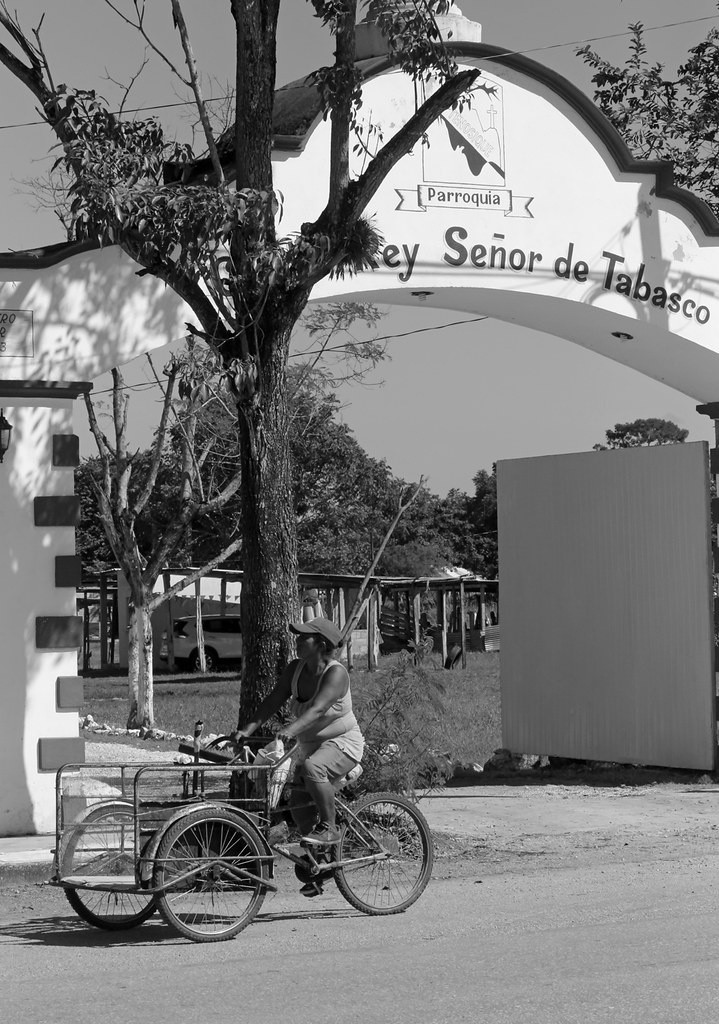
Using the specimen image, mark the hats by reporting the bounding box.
[288,617,343,650]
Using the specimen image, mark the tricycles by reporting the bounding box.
[45,734,435,943]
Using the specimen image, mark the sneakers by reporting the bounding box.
[300,821,342,845]
[299,880,323,894]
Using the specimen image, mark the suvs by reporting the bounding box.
[160,614,242,673]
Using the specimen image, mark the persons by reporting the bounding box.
[448,605,467,633]
[475,609,497,629]
[230,617,364,894]
